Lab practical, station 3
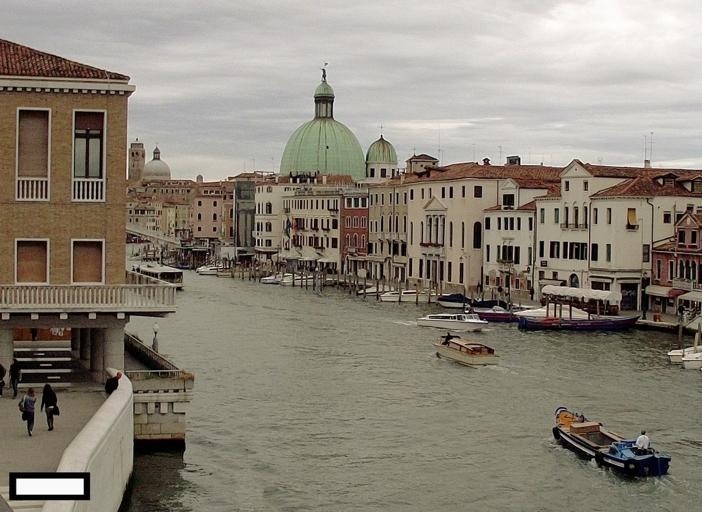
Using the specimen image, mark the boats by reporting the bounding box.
[469,298,513,311]
[513,303,593,321]
[552,406,671,477]
[517,284,642,333]
[437,292,478,312]
[125,233,439,313]
[682,351,702,370]
[431,336,500,365]
[416,311,489,333]
[667,344,702,364]
[473,306,517,323]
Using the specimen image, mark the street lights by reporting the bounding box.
[151,323,159,354]
[642,271,647,320]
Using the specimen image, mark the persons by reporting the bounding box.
[677,304,687,322]
[29,326,38,341]
[632,429,650,455]
[105,373,122,394]
[440,332,453,347]
[18,387,36,436]
[40,383,58,431]
[0,363,7,398]
[9,359,20,399]
